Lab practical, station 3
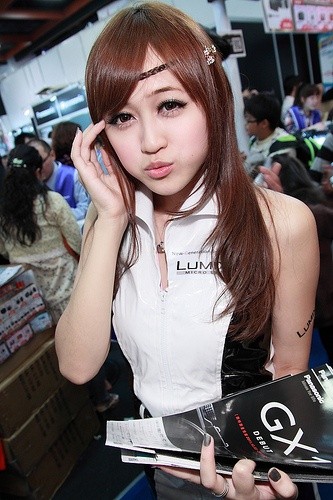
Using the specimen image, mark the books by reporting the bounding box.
[105,363,333,483]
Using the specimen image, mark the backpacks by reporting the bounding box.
[269,130,321,172]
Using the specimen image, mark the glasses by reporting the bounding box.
[244,117,259,125]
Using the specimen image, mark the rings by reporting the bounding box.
[212,476,229,498]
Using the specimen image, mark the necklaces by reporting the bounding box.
[155,222,166,253]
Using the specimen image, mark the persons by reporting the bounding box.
[238,74,333,366]
[0,145,141,420]
[0,122,109,233]
[55,0,320,500]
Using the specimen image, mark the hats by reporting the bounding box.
[8,145,43,173]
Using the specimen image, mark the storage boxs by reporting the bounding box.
[0,326,100,500]
